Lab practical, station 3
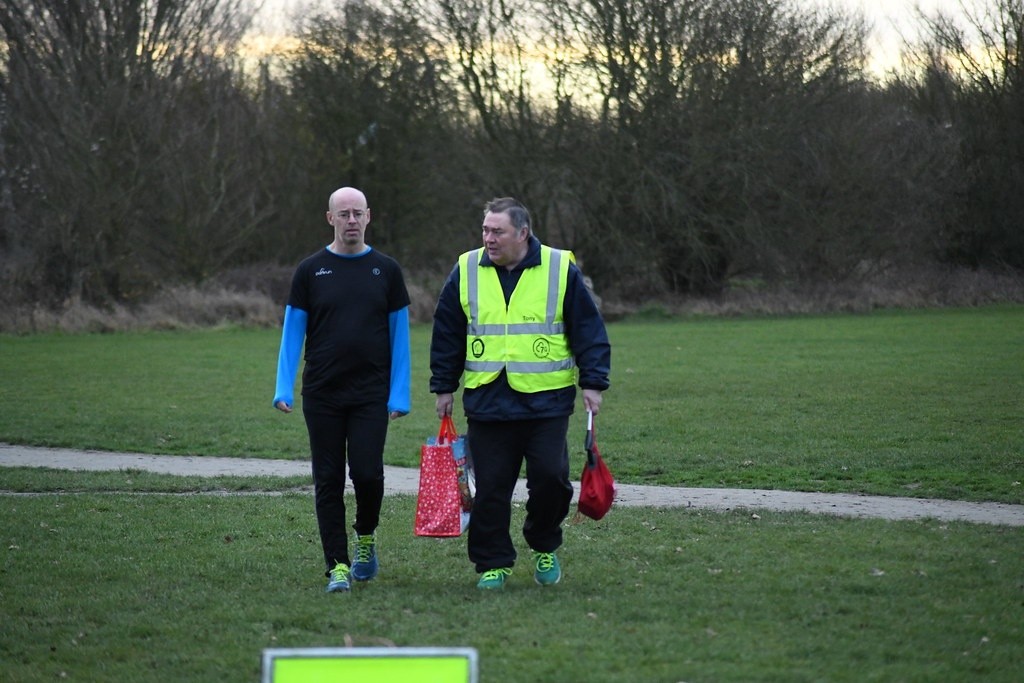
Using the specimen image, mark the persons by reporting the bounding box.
[429,198,612,587]
[275,187,411,592]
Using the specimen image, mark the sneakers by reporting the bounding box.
[477,567,511,592]
[528,549,562,585]
[351,527,378,581]
[326,564,352,592]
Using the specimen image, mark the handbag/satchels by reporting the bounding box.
[415,413,476,538]
[576,413,617,522]
